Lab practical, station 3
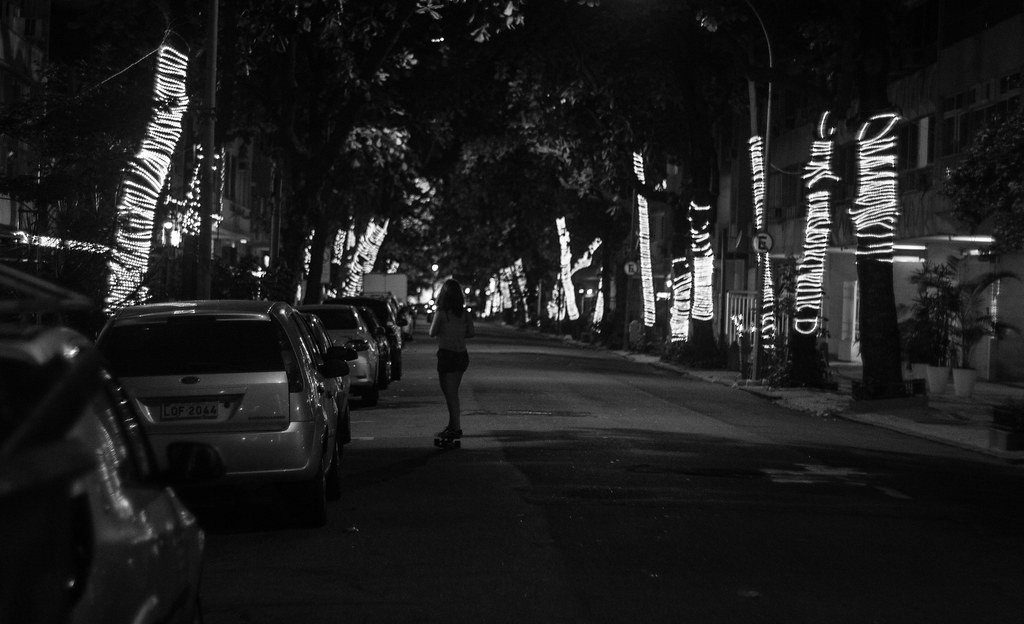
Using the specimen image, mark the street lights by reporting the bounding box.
[593,108,639,353]
[697,1,774,380]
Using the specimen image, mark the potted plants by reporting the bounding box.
[901,257,1021,400]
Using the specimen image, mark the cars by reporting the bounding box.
[0,317,214,624]
[288,290,418,466]
[91,296,352,529]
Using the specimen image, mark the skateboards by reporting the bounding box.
[433,431,462,449]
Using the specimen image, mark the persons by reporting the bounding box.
[428,280,475,438]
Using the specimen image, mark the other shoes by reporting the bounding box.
[434,427,461,442]
